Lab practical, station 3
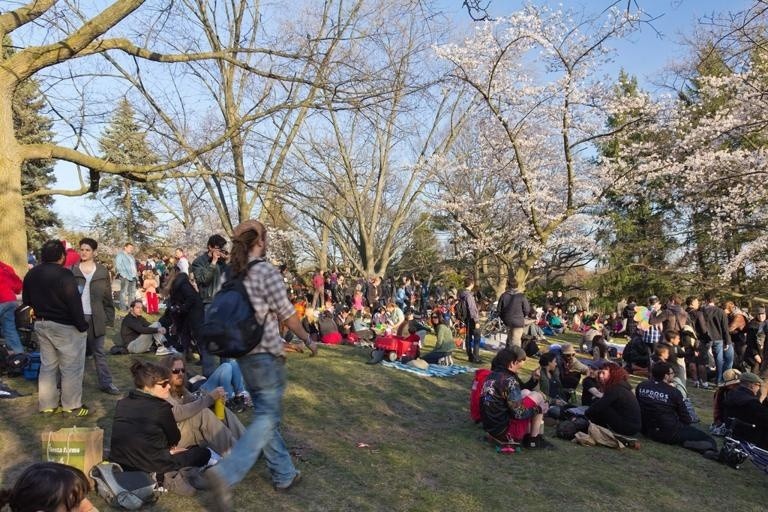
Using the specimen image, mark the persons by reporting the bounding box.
[2,214,768,512]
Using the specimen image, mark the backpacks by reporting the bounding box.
[88,459,158,510]
[454,295,468,321]
[199,260,273,358]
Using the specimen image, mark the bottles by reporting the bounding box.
[214,386,225,420]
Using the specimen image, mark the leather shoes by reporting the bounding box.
[706,451,725,463]
[683,440,714,450]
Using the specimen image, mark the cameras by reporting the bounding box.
[226,395,245,412]
[211,248,228,263]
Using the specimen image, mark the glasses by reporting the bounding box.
[748,381,763,388]
[566,354,574,357]
[668,372,675,376]
[172,368,185,374]
[154,382,169,388]
[431,316,438,320]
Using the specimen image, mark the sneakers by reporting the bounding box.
[700,380,710,387]
[525,433,558,451]
[156,346,181,356]
[274,470,302,492]
[63,405,98,419]
[102,383,123,395]
[162,468,232,511]
[44,404,63,415]
[694,383,699,388]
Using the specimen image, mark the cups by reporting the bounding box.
[539,420,544,434]
[719,436,740,463]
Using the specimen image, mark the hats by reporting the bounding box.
[741,373,765,381]
[562,344,577,353]
[756,308,766,315]
[718,369,744,387]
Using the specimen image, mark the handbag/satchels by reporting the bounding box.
[21,351,42,380]
[683,397,702,423]
[109,344,128,356]
[551,316,562,328]
[555,417,589,441]
[6,352,27,377]
[321,332,344,344]
[681,324,700,350]
[41,426,104,491]
